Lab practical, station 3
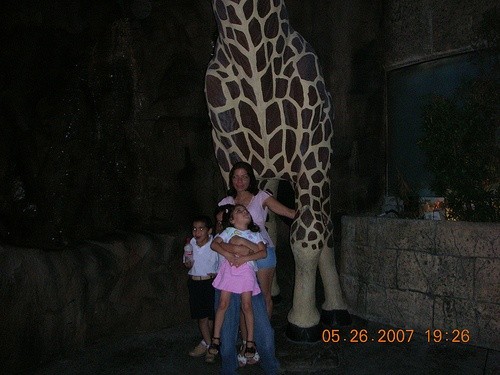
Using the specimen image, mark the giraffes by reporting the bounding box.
[204,0,349,329]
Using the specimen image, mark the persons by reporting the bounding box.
[207,204,268,359]
[181,215,220,363]
[217,162,296,367]
[211,205,285,375]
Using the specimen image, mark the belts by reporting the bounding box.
[190,274,215,280]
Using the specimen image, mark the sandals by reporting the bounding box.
[244,340,256,359]
[209,336,221,355]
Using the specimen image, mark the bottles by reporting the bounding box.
[184,238,193,263]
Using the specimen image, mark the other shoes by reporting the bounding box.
[204,346,219,363]
[248,350,262,366]
[190,340,208,356]
[237,346,248,367]
